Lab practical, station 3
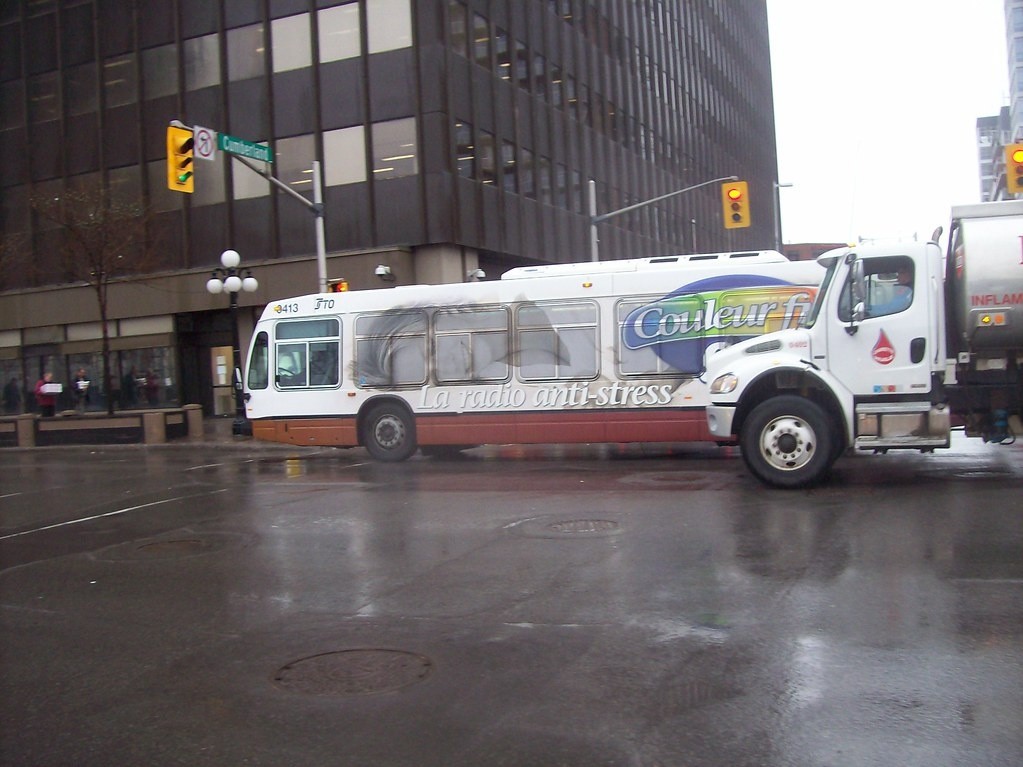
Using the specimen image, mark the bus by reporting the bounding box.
[235,251,843,464]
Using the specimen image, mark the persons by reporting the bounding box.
[72,368,90,410]
[35,371,56,417]
[2,376,22,414]
[128,363,139,410]
[865,258,914,318]
[144,367,161,409]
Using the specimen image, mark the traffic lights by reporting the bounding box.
[1005,142,1022,195]
[721,180,754,231]
[167,121,199,194]
[330,279,342,293]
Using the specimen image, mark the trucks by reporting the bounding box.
[701,202,1023,489]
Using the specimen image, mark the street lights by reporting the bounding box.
[773,181,793,252]
[207,249,259,436]
